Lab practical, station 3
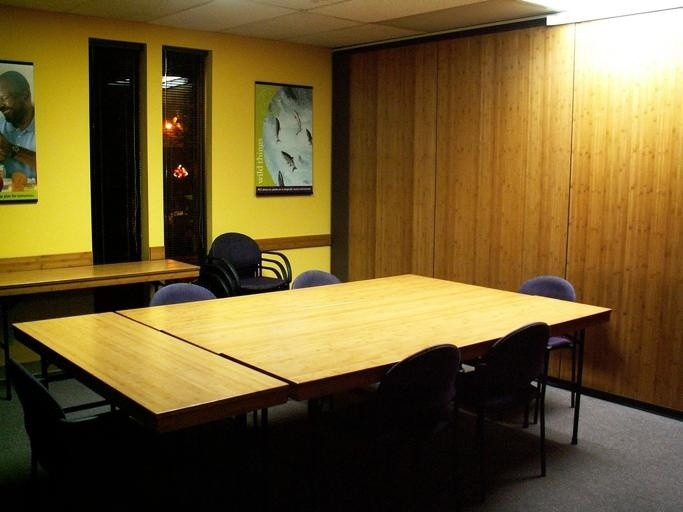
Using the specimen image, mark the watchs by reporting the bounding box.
[6,144,20,158]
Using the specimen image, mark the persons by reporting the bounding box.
[0,71,36,180]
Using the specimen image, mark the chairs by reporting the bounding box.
[5,354,152,512]
[331,344,459,507]
[198,233,291,298]
[515,276,578,425]
[150,282,259,428]
[451,322,549,478]
[292,270,340,290]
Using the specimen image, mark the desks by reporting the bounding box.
[12,311,291,509]
[117,268,612,512]
[0,258,200,400]
